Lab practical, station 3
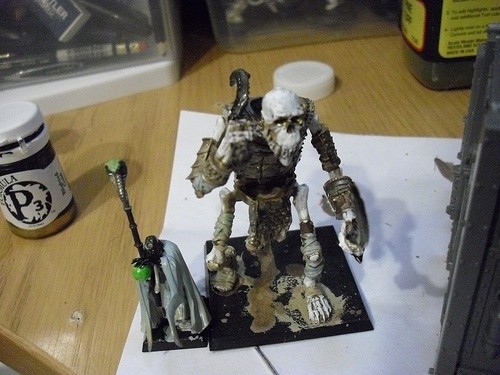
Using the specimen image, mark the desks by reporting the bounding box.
[1,40,500,375]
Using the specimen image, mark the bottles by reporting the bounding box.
[398,0,499,93]
[1,99,77,238]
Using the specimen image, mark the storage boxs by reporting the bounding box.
[400,0,500,93]
[205,0,403,56]
[1,0,179,120]
